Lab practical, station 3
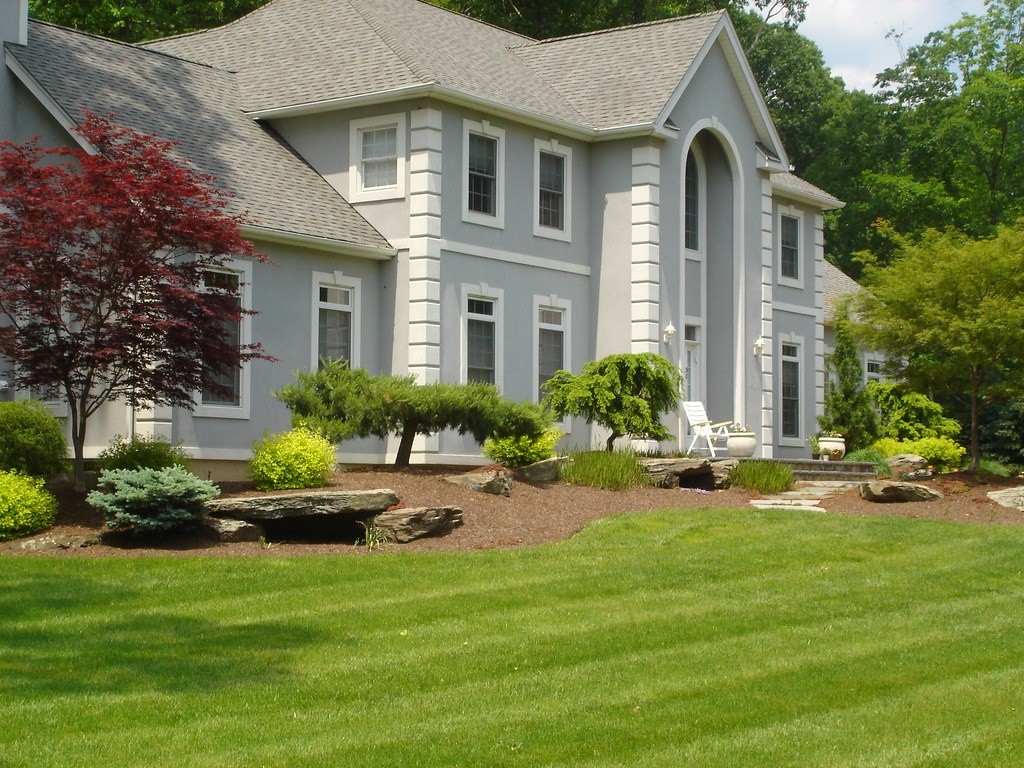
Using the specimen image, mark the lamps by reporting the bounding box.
[663,321,677,345]
[753,335,765,358]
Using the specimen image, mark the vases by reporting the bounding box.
[818,438,846,460]
[727,432,758,457]
[822,455,830,461]
[811,454,820,460]
[630,440,659,457]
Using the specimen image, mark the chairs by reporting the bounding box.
[682,401,733,459]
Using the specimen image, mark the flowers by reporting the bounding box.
[729,423,753,432]
[806,430,844,455]
